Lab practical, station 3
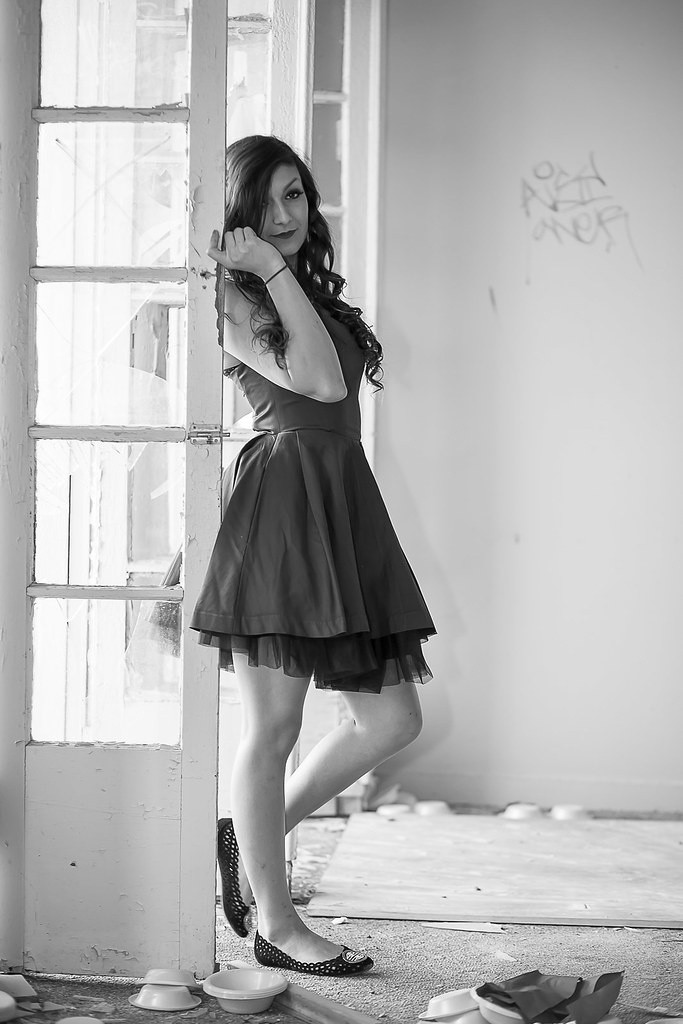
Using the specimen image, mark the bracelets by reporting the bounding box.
[264,264,289,285]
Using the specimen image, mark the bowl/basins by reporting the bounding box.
[128,984,200,1011]
[203,968,288,1014]
[138,968,202,991]
[417,986,548,1024]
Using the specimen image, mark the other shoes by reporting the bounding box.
[217,817,251,938]
[253,929,375,977]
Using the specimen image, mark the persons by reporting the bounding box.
[151,134,438,979]
